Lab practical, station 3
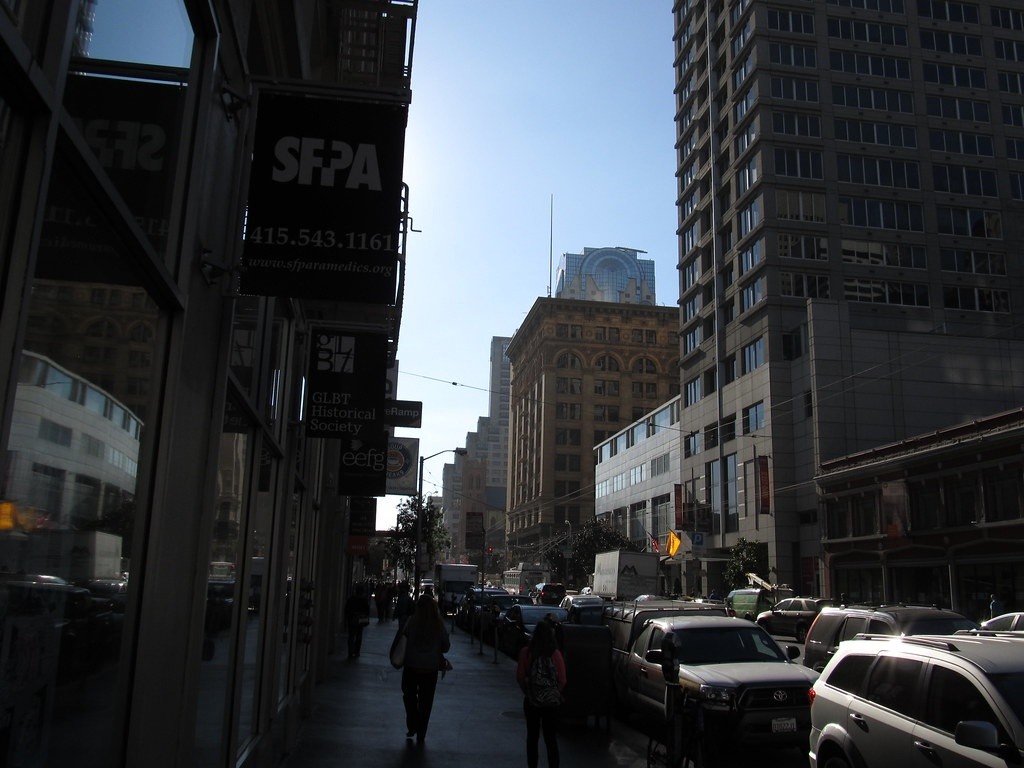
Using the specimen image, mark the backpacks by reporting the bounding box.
[527,651,560,709]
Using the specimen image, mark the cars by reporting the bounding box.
[408,577,434,596]
[969,611,1024,636]
[457,582,797,660]
[202,556,266,637]
[0,573,128,704]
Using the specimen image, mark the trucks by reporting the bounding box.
[433,562,479,619]
[587,549,661,602]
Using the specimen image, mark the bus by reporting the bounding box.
[503,563,550,597]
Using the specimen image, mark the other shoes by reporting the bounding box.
[406,728,416,740]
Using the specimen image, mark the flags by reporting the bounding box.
[649,531,681,558]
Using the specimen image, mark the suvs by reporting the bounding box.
[755,596,842,644]
[807,629,1024,768]
[804,601,997,674]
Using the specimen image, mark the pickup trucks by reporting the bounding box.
[601,599,821,768]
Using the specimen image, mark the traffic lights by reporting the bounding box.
[488,547,492,556]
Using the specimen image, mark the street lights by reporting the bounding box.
[414,447,467,602]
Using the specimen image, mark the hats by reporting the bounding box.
[544,613,556,622]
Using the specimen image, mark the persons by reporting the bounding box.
[841,593,849,602]
[991,593,1004,619]
[345,578,451,743]
[658,589,736,618]
[516,621,565,768]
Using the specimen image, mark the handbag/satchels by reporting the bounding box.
[391,616,412,670]
